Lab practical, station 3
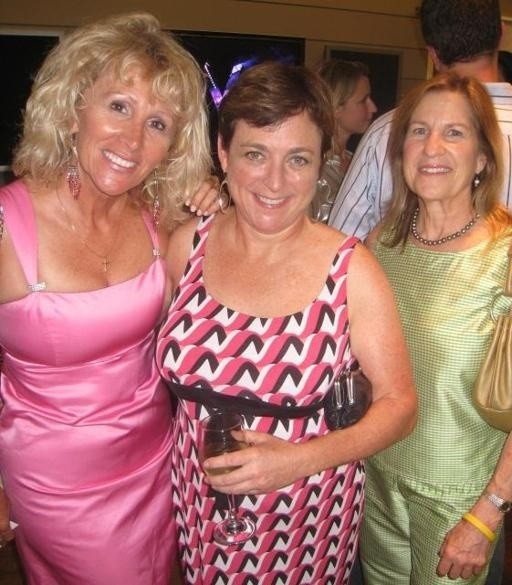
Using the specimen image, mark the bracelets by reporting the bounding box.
[462,514,496,542]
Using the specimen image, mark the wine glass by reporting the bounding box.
[196,409,257,545]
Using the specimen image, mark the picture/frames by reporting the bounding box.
[324,44,404,152]
[165,29,305,82]
[426,16,512,81]
[0,29,68,172]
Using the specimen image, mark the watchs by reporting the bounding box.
[482,490,512,514]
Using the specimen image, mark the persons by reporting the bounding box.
[153,60,419,585]
[326,0,511,247]
[1,9,230,585]
[303,57,379,225]
[356,71,511,585]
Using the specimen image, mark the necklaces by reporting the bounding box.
[411,208,481,245]
[55,173,127,271]
